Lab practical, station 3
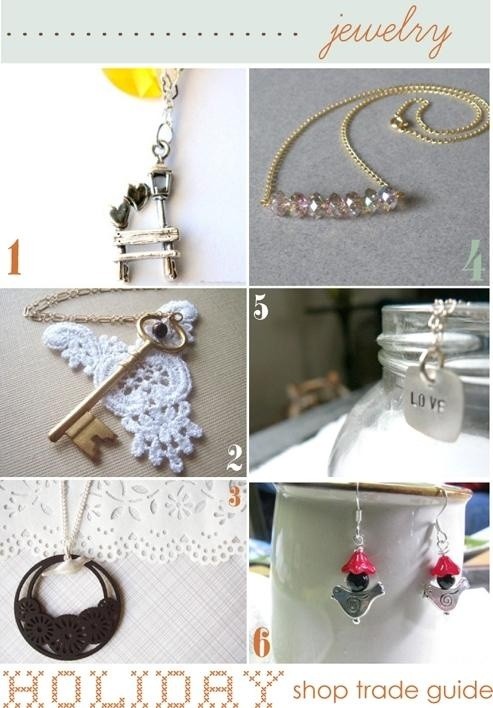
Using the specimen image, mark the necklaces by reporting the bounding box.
[403,298,466,445]
[15,480,123,663]
[261,85,490,221]
[107,68,182,283]
[23,288,189,463]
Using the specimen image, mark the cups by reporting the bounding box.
[268,482,472,663]
